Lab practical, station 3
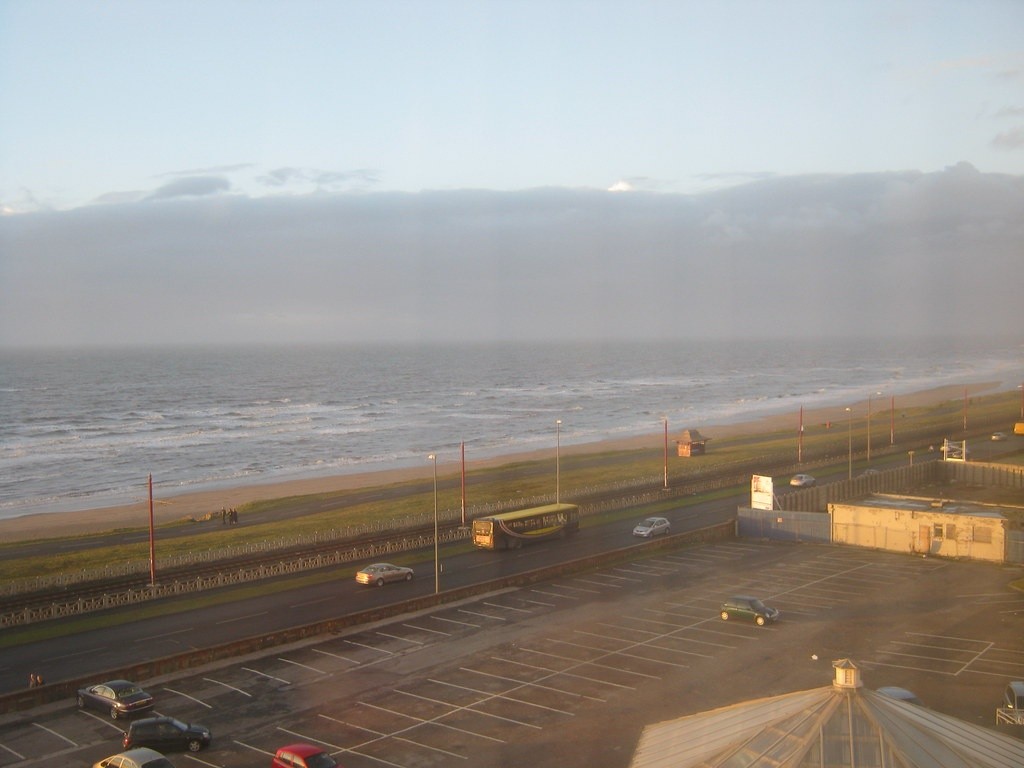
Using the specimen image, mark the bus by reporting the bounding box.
[472,503,579,552]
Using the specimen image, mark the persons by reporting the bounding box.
[221,507,238,525]
[30,673,48,696]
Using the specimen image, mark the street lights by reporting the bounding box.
[868,391,883,462]
[429,455,439,594]
[846,408,852,480]
[556,420,563,504]
[1017,385,1024,419]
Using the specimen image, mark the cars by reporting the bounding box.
[1001,681,1024,725]
[790,474,815,489]
[355,563,414,588]
[877,686,929,709]
[93,747,177,768]
[940,446,968,456]
[633,517,670,540]
[123,716,211,753]
[76,679,156,721]
[992,432,1007,441]
[719,595,780,626]
[273,741,347,768]
